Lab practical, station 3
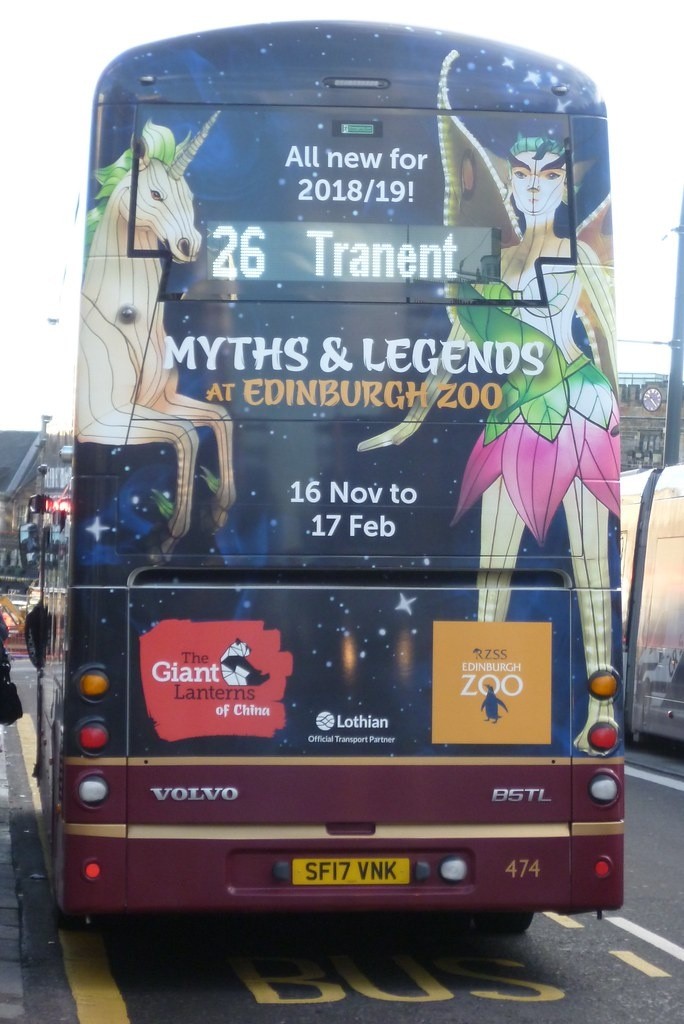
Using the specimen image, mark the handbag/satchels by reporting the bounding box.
[0,661,23,726]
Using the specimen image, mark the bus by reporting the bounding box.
[35,18,628,951]
